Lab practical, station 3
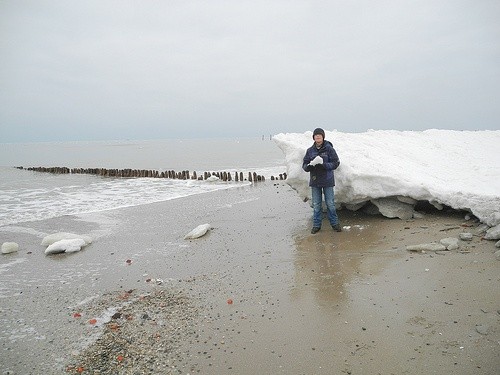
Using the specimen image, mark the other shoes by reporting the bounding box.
[311,227,320,233]
[333,224,341,232]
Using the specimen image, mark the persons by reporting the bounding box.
[302,128,342,234]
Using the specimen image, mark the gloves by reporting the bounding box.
[307,164,315,170]
[315,164,324,170]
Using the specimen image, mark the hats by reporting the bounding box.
[313,128,325,138]
